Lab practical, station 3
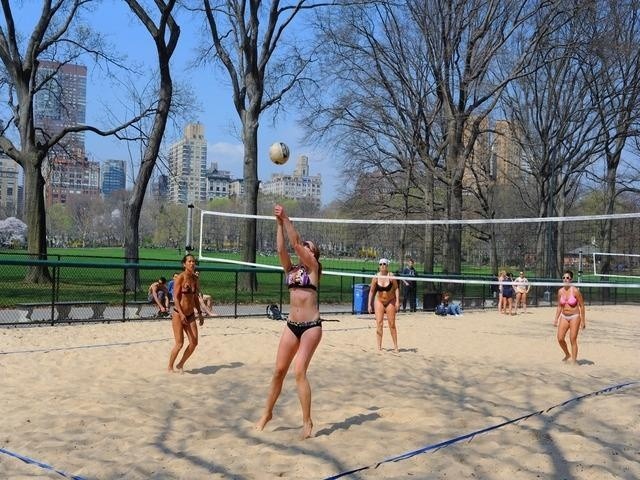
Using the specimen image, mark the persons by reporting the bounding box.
[167,252,205,375]
[512,271,530,315]
[195,270,218,317]
[251,201,325,443]
[367,256,402,354]
[489,269,516,318]
[440,292,463,318]
[401,259,417,314]
[553,269,586,366]
[168,272,179,301]
[147,275,171,317]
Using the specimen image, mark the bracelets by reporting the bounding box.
[197,310,203,314]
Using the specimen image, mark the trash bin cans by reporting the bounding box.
[422,292,442,312]
[353,283,371,315]
[175,248,181,255]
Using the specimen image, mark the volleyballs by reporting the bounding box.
[268,141,288,165]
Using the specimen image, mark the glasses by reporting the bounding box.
[300,241,314,252]
[379,263,387,266]
[562,276,571,280]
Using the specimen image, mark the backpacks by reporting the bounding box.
[266,303,282,320]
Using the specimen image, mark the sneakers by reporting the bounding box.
[157,309,170,318]
